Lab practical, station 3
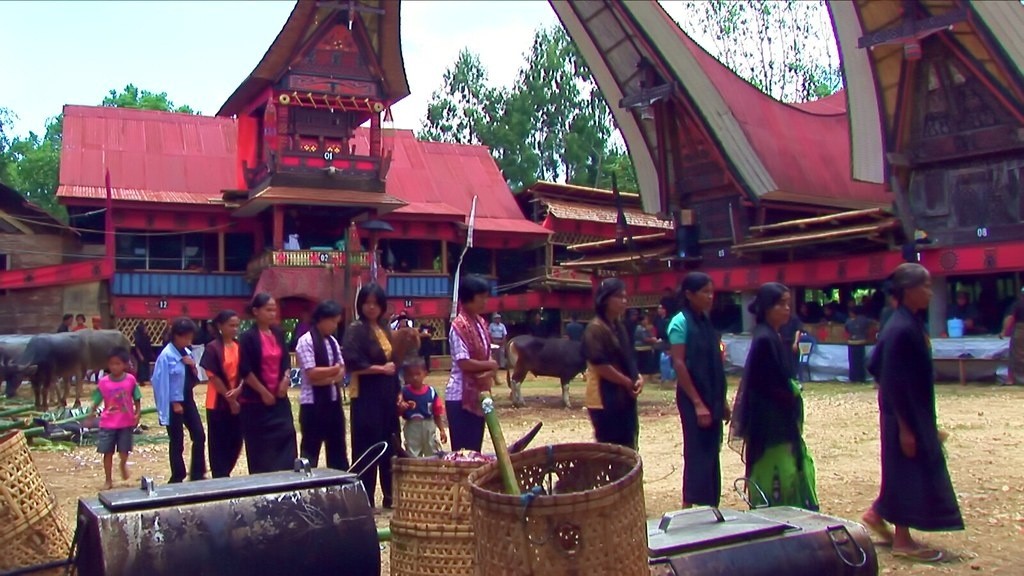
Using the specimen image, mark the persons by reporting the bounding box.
[860,260,966,562]
[294,299,351,470]
[668,271,731,510]
[198,309,244,478]
[342,282,401,506]
[150,315,209,484]
[55,285,1024,386]
[237,291,300,474]
[446,272,500,455]
[578,277,644,451]
[728,282,818,513]
[396,353,448,458]
[82,345,142,491]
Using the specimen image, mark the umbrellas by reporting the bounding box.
[359,220,394,240]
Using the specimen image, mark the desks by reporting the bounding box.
[929,336,1024,386]
[798,342,882,383]
[720,332,755,376]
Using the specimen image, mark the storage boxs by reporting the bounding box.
[69,440,392,576]
[646,477,879,576]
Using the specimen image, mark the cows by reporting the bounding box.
[0,333,62,407]
[505,334,589,408]
[2,329,145,414]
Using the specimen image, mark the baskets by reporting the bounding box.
[389,457,491,576]
[466,441,651,576]
[0,428,77,576]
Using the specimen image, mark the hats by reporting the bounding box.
[494,314,502,318]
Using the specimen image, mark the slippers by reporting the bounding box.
[869,535,894,547]
[890,543,943,563]
